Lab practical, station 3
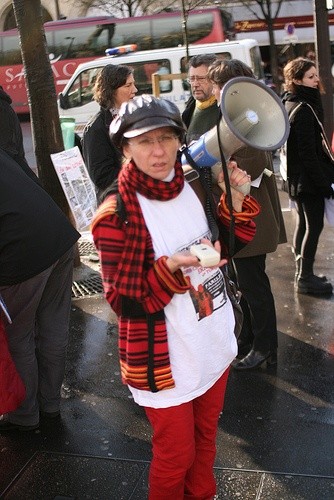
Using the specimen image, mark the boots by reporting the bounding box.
[295,254,333,292]
[295,255,327,287]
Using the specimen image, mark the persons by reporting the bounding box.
[0,146,82,428]
[82,65,138,203]
[278,56,334,296]
[91,94,240,500]
[182,54,221,126]
[150,63,170,91]
[207,57,288,371]
[82,76,96,92]
[0,85,28,154]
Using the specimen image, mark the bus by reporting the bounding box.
[0,7,237,120]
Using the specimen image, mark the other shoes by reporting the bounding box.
[39,412,59,417]
[0,414,40,432]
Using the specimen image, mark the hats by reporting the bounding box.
[108,95,187,155]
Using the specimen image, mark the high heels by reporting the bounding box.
[235,347,278,370]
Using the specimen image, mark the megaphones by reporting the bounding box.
[181,76,290,196]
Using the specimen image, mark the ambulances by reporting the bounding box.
[56,39,270,157]
